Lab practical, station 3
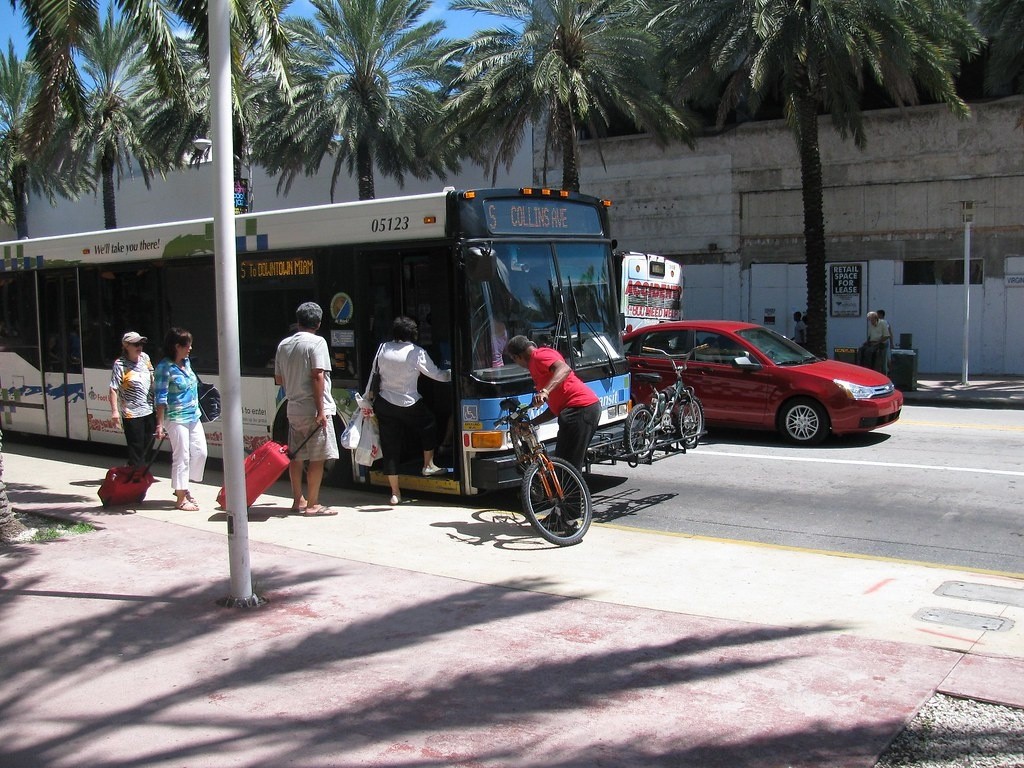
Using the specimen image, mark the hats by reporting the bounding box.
[122,332,148,344]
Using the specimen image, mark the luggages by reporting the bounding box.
[98,428,167,506]
[215,420,323,515]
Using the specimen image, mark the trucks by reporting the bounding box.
[526,251,685,349]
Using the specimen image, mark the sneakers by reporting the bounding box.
[537,516,577,535]
[389,494,402,505]
[421,466,448,477]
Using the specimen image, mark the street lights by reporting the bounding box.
[194,139,255,214]
[949,200,988,384]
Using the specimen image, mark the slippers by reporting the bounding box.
[289,502,338,516]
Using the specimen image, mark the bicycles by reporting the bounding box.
[493,398,593,546]
[622,343,710,464]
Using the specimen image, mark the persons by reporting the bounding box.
[274,302,339,516]
[154,327,207,510]
[857,310,893,377]
[364,317,451,505]
[473,295,508,369]
[646,333,776,364]
[506,336,602,531]
[790,312,808,351]
[109,332,158,466]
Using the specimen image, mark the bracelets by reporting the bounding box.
[156,424,165,429]
[539,388,550,396]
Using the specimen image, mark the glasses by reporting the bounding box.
[132,342,146,347]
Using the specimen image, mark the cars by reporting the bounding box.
[621,320,904,449]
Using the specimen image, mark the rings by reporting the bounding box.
[538,397,540,401]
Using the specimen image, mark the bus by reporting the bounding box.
[0,187,649,497]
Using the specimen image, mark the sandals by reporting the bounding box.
[173,492,199,512]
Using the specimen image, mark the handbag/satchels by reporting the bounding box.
[196,382,223,426]
[355,391,384,467]
[341,390,370,449]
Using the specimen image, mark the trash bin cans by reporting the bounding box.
[889,348,919,391]
